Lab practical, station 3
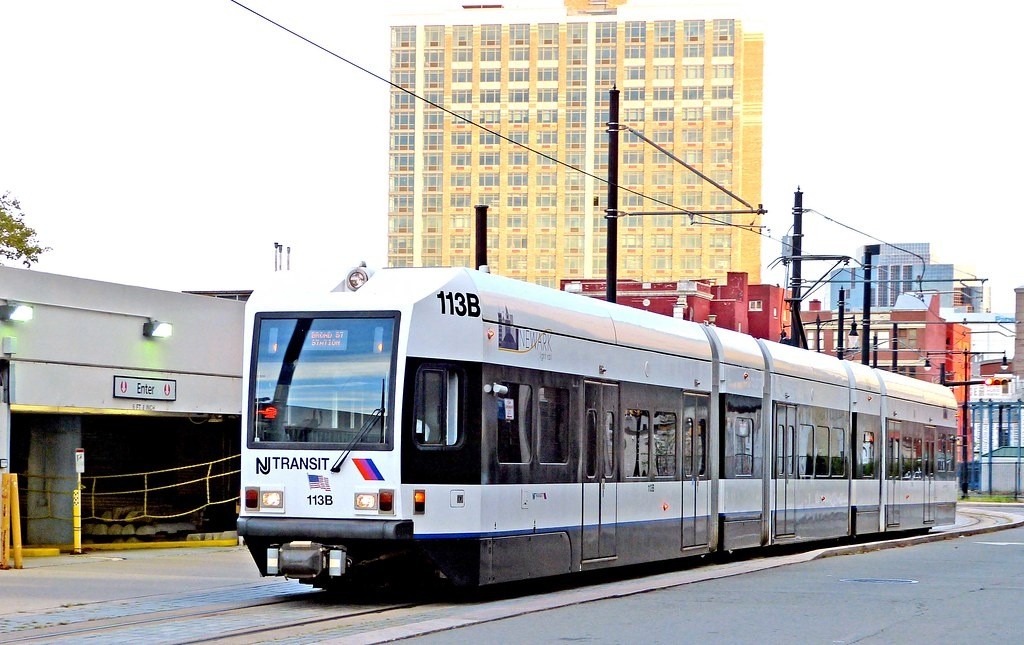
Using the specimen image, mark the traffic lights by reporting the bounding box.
[985,378,1008,385]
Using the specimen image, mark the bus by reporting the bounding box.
[235,257,961,610]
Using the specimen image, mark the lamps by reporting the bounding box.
[144,321,173,338]
[0,305,34,322]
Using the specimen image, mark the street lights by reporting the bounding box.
[780,314,859,353]
[923,350,1008,498]
